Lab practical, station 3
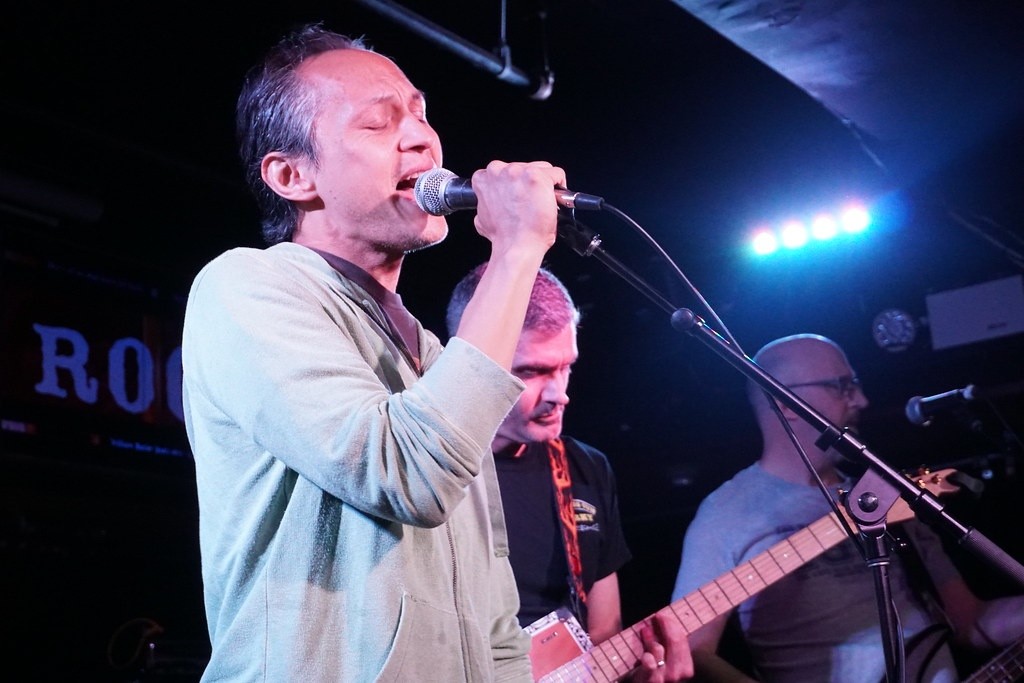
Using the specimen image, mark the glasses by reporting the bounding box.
[780,376,859,397]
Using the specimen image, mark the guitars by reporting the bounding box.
[960,636,1024,683]
[523,466,961,683]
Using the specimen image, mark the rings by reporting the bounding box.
[658,660,665,666]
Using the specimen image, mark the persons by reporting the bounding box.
[180,27,568,683]
[447,259,695,683]
[671,333,1024,683]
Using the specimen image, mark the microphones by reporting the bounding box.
[905,385,974,425]
[414,168,604,216]
